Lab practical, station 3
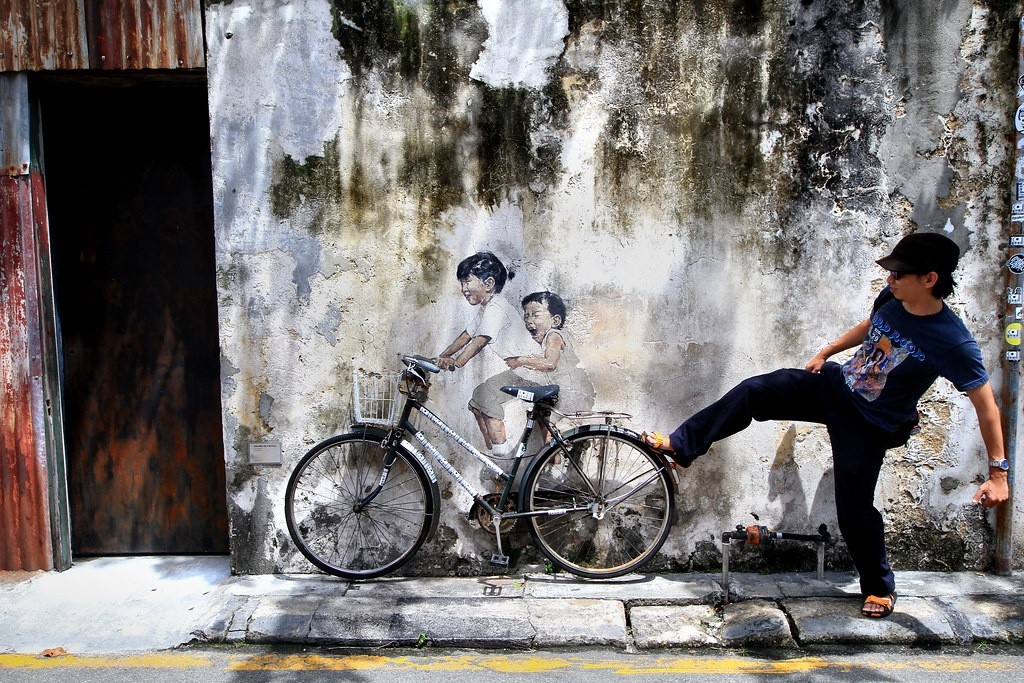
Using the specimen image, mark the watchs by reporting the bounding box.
[987,458,1011,472]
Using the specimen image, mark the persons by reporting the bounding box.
[637,228,1013,619]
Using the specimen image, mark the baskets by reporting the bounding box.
[353,368,402,426]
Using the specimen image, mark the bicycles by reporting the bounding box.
[284,354,680,581]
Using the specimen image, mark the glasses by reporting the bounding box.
[890,270,904,280]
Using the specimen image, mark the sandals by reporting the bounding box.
[861,591,897,618]
[642,430,692,469]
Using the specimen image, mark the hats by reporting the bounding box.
[875,233,960,274]
